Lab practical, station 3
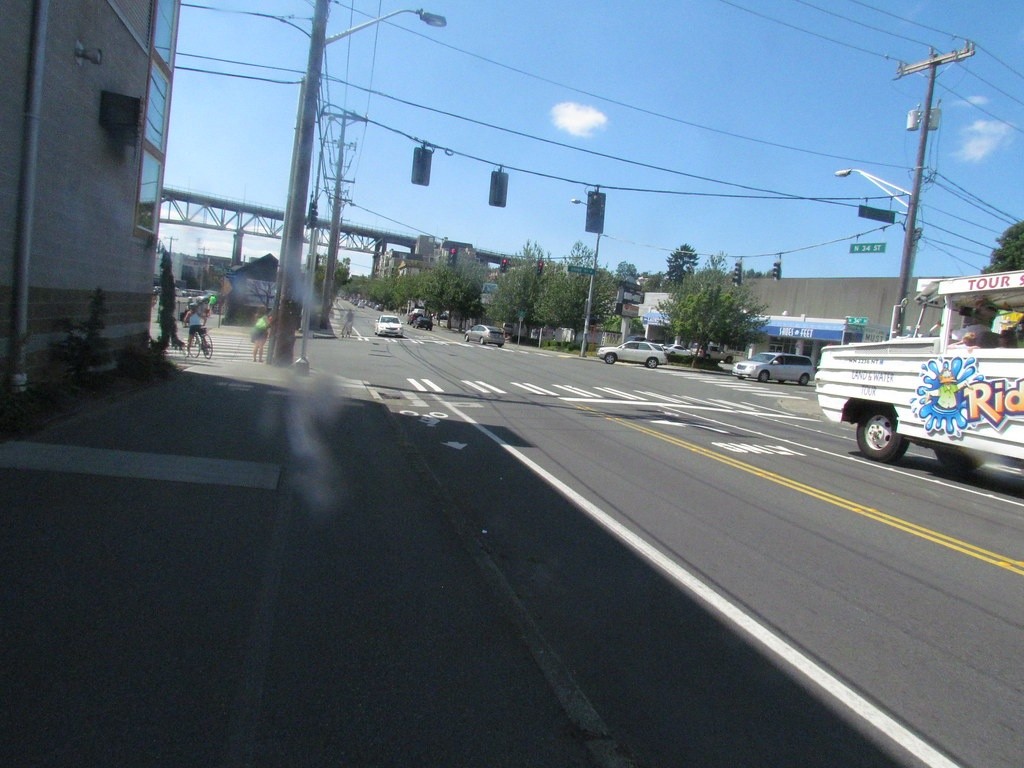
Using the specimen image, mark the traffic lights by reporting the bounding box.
[308,202,318,223]
[447,248,458,267]
[732,261,742,287]
[537,262,544,274]
[772,262,781,280]
[501,258,508,273]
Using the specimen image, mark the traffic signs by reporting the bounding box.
[850,243,886,253]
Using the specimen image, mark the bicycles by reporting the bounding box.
[183,325,213,359]
[342,320,351,338]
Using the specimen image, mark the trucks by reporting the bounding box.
[815,269,1024,472]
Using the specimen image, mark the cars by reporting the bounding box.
[597,342,668,369]
[374,315,404,337]
[407,309,449,331]
[464,325,505,348]
[663,343,734,364]
[340,295,383,311]
[153,286,217,297]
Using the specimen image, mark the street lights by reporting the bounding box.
[833,168,919,338]
[571,199,600,357]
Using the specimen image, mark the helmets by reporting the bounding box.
[190,303,198,306]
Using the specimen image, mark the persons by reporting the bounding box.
[253,305,268,363]
[341,309,354,335]
[930,294,1024,349]
[184,298,211,358]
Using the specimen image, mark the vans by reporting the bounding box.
[732,353,814,386]
